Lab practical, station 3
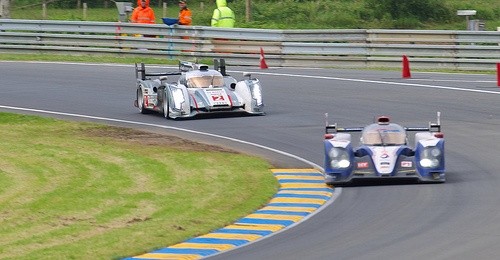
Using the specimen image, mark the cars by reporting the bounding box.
[320,109,448,187]
[132,57,266,120]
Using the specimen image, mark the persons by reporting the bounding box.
[177,0,192,53]
[210,0,236,54]
[132,0,156,51]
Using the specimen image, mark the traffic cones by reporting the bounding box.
[401,55,412,78]
[258,47,268,70]
